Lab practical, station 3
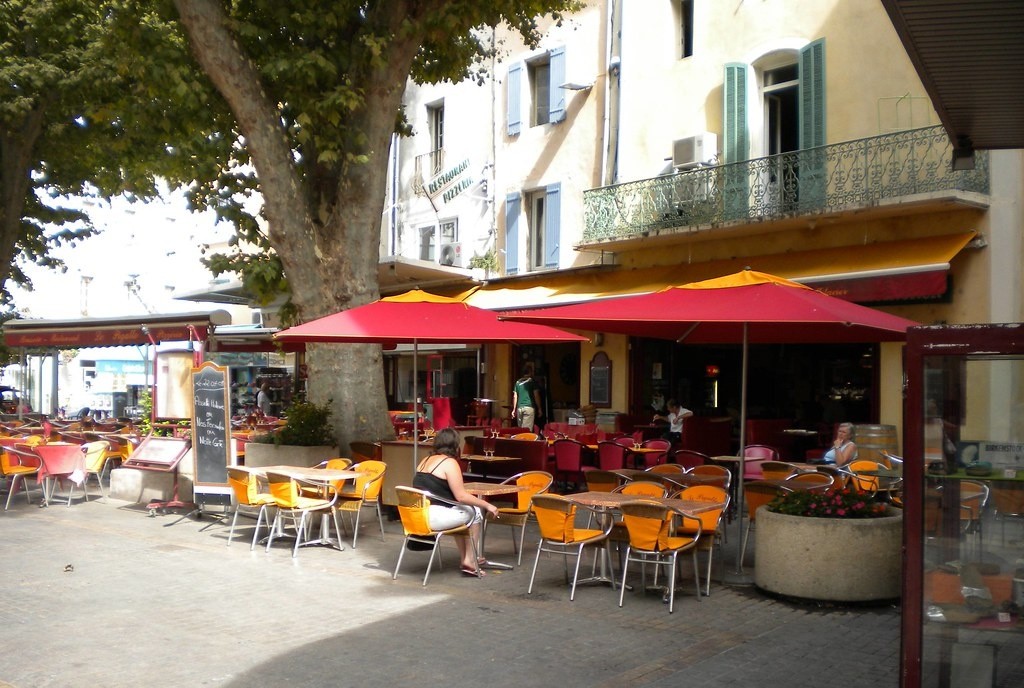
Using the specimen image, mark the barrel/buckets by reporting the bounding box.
[854,424,898,470]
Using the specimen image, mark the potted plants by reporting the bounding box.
[244,391,340,467]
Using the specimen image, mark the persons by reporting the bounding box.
[824,422,858,467]
[925,400,956,479]
[254,378,276,417]
[648,388,669,424]
[414,428,499,576]
[653,397,693,447]
[511,364,543,433]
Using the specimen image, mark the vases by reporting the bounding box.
[755,504,902,603]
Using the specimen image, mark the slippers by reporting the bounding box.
[462,567,486,576]
[477,557,486,564]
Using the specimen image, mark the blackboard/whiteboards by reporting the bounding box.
[189,360,231,488]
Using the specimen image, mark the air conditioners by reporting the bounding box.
[438,242,466,267]
[671,170,717,207]
[672,132,718,167]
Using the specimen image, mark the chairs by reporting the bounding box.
[225,457,389,558]
[0,416,138,511]
[393,414,1024,613]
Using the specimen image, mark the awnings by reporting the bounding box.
[466,233,978,303]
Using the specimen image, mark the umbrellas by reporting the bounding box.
[498,267,922,573]
[270,288,588,480]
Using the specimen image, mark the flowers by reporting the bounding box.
[775,489,896,518]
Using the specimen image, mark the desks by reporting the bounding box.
[564,490,723,516]
[709,453,764,464]
[248,462,362,482]
[464,481,530,496]
[459,447,522,462]
[545,438,666,453]
[381,428,436,505]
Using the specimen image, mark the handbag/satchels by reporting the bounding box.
[407,535,436,551]
[807,458,826,465]
